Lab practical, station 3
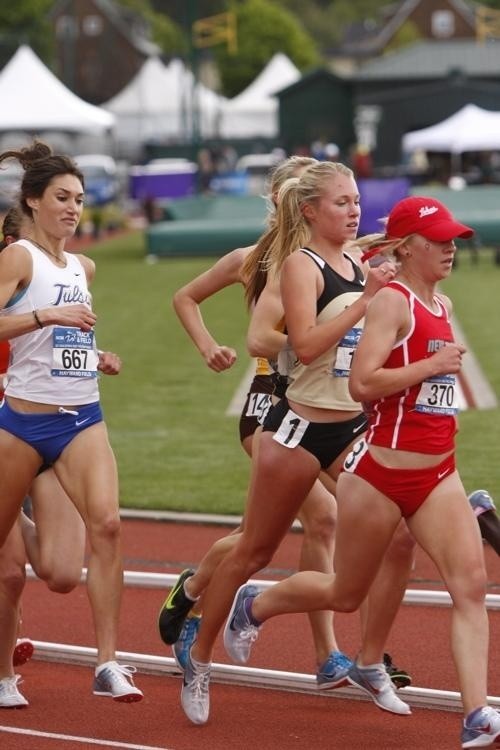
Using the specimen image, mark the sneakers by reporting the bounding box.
[178,648,213,726]
[462,706,500,748]
[172,608,202,675]
[223,585,265,665]
[93,664,145,703]
[0,673,28,709]
[158,568,200,646]
[469,490,495,517]
[315,649,412,716]
[13,639,34,668]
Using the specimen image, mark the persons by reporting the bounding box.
[3,141,500,243]
[0,143,149,706]
[173,233,368,694]
[224,197,500,749]
[0,204,75,670]
[2,346,121,708]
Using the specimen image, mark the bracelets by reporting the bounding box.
[31,310,44,328]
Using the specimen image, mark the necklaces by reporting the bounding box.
[22,236,70,270]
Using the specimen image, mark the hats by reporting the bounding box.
[384,196,475,244]
